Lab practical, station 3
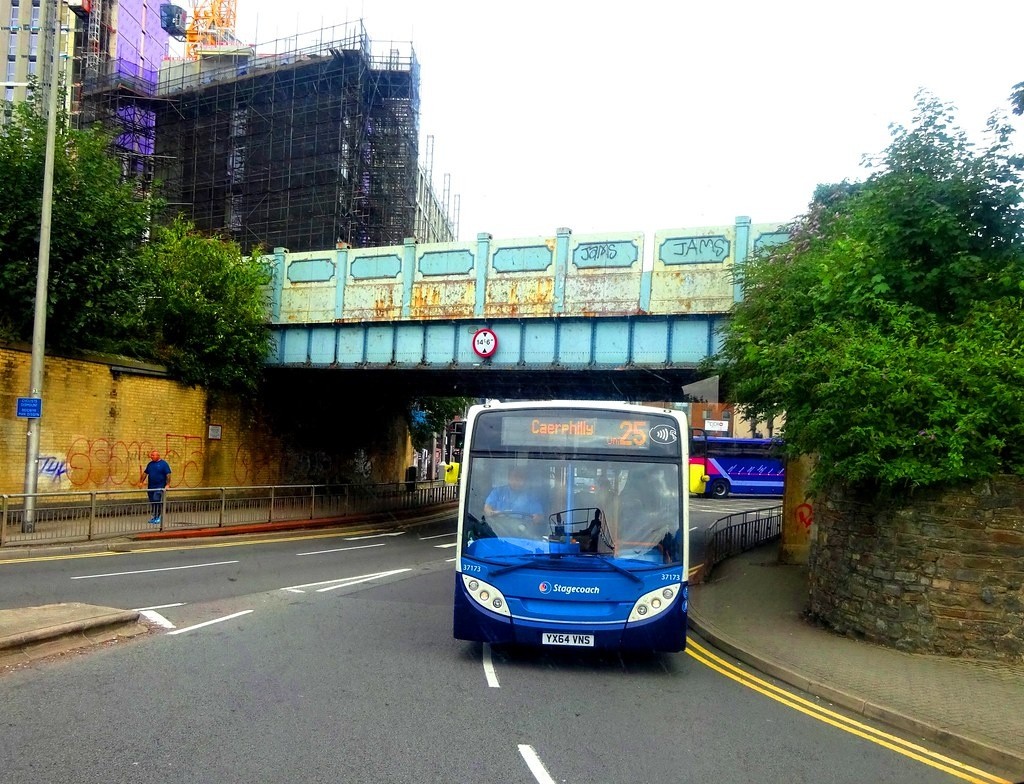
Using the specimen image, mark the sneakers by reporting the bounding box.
[153,517,160,524]
[148,518,155,523]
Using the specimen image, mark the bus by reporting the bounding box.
[690,436,785,499]
[443,401,710,654]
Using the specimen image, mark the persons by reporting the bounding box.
[484,469,545,524]
[141,452,171,523]
